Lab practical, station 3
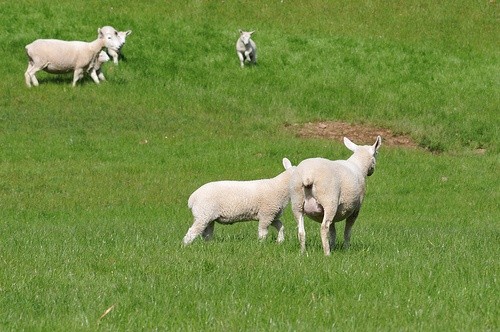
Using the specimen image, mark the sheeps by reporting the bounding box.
[98,25,132,66]
[236,29,257,70]
[24,27,119,89]
[288,135,383,258]
[179,157,296,250]
